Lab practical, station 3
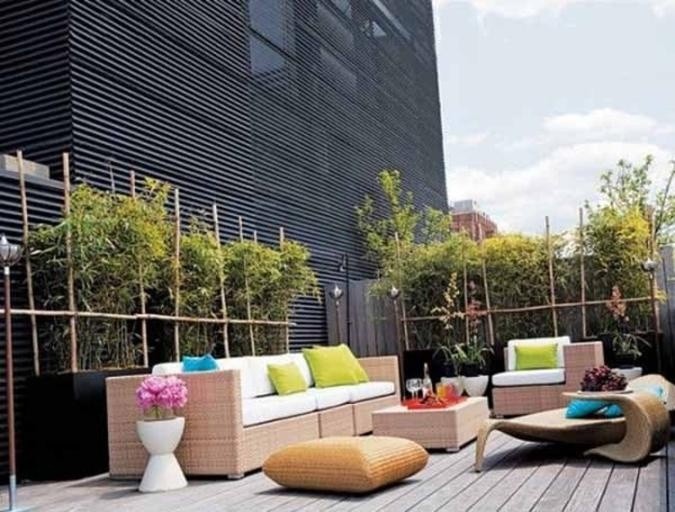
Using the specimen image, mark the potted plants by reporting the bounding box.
[609,330,649,379]
[350,168,509,408]
[25,178,154,483]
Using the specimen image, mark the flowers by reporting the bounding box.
[136,376,188,421]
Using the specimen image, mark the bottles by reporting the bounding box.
[422,363,432,400]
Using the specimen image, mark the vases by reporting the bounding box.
[132,416,191,490]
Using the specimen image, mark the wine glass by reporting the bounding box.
[406,379,418,401]
[412,378,422,399]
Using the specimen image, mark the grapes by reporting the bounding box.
[579,362,629,391]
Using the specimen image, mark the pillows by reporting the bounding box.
[513,344,558,371]
[569,385,661,418]
[183,355,219,372]
[268,342,368,393]
[259,433,428,493]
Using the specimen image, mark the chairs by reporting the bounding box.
[488,336,602,414]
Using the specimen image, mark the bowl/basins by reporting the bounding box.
[442,376,464,398]
[465,375,489,397]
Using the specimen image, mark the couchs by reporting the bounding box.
[103,352,403,482]
[472,374,673,479]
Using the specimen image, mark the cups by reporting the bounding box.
[437,383,449,399]
[444,384,457,398]
